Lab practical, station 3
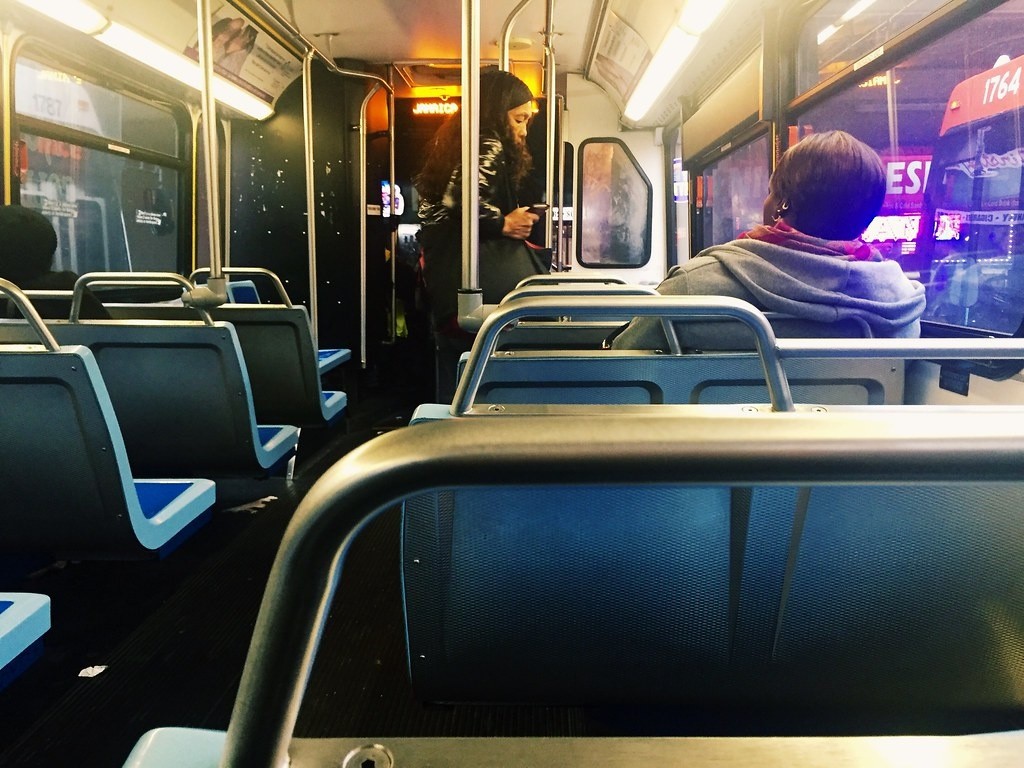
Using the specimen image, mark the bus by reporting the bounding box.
[909,54,1024,338]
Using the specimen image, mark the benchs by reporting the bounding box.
[0,265,1024,768]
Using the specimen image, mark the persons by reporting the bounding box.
[337,223,435,411]
[607,130,928,350]
[415,70,539,395]
[0,204,112,321]
[931,234,980,321]
